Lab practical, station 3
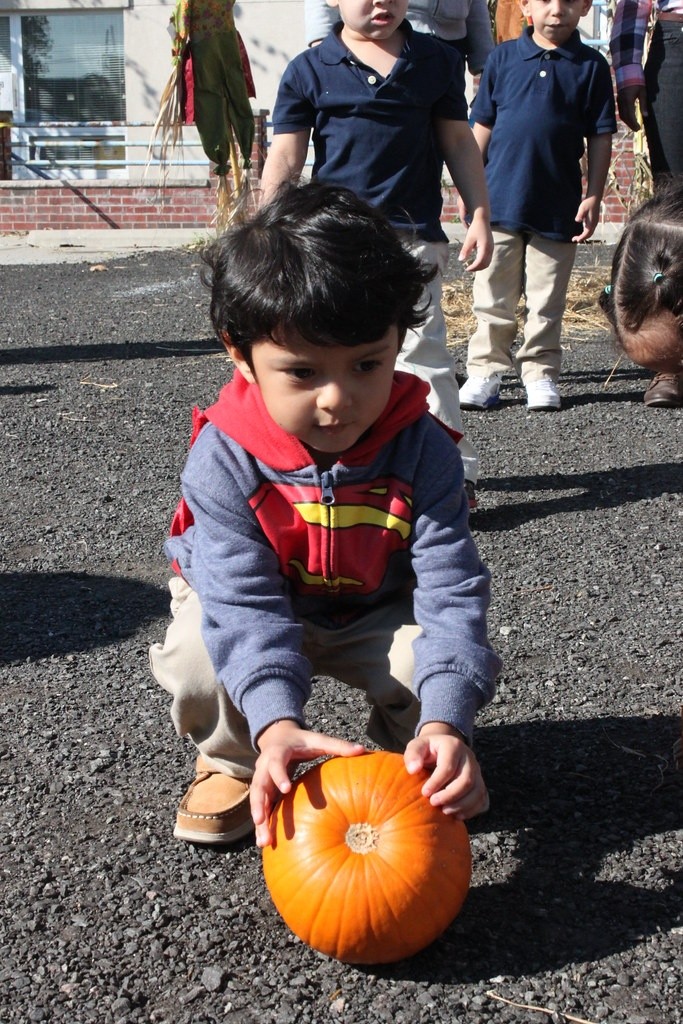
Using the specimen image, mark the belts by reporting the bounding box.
[656,12,683,23]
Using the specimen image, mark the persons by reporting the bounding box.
[594,1,682,193]
[249,1,492,508]
[461,1,614,418]
[149,183,502,848]
[601,194,681,377]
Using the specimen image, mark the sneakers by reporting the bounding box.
[526,379,562,413]
[459,378,501,410]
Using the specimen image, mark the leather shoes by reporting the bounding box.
[644,372,683,408]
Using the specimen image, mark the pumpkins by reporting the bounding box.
[260,750,472,963]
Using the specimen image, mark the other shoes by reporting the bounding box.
[463,480,477,509]
[174,754,255,840]
